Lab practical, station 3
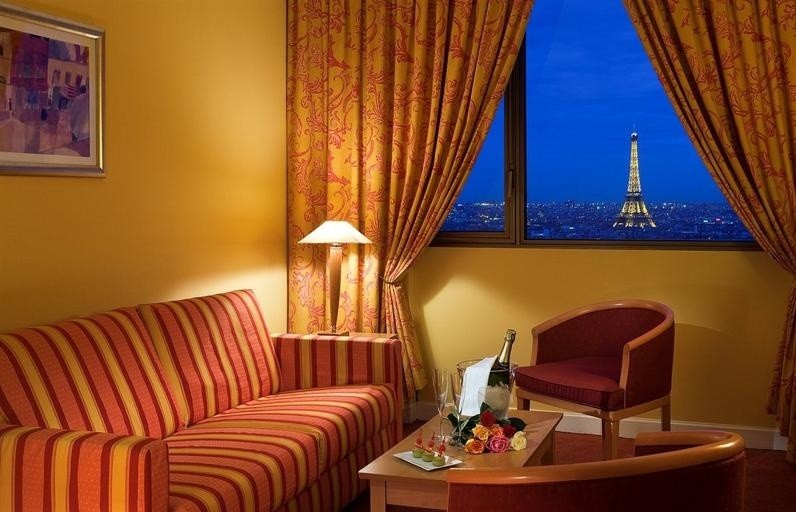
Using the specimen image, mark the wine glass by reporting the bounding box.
[451,375,464,432]
[431,367,450,440]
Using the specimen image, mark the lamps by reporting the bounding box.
[296,220,373,336]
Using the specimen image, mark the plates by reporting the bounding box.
[393,448,465,472]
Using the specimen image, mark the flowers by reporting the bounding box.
[444,403,527,455]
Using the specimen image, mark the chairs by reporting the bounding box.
[448,431,745,512]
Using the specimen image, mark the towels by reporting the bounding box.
[458,355,498,416]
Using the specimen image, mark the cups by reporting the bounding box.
[456,359,519,424]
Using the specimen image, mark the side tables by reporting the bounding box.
[306,332,399,340]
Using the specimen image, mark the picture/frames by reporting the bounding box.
[0,2,106,178]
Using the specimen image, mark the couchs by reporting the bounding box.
[0,289,404,512]
[515,298,675,435]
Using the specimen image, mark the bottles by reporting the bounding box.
[484,329,517,420]
